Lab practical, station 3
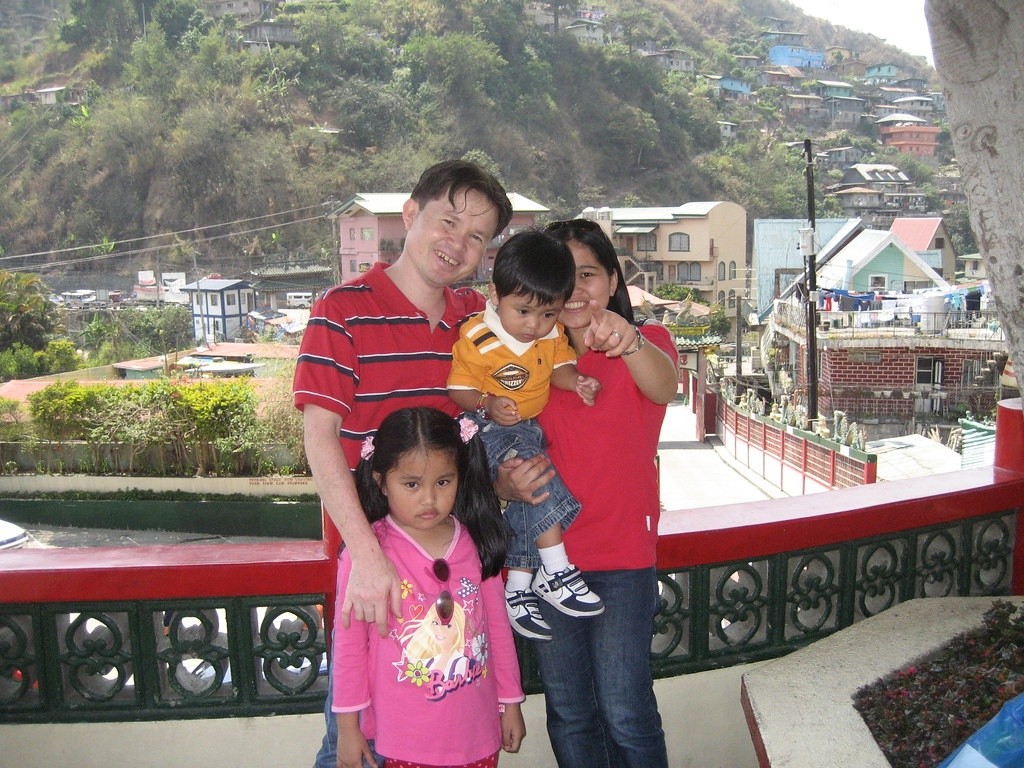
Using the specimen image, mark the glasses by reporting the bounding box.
[424,558,454,625]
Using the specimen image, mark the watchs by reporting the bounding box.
[620,325,644,356]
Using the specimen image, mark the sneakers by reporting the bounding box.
[530,564,605,618]
[503,582,552,642]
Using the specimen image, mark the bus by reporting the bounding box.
[286,292,320,309]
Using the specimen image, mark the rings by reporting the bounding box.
[610,332,622,340]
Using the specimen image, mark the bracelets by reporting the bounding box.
[476,392,495,422]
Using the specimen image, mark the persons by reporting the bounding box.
[447,229,606,643]
[482,219,680,768]
[292,159,514,766]
[330,406,529,767]
